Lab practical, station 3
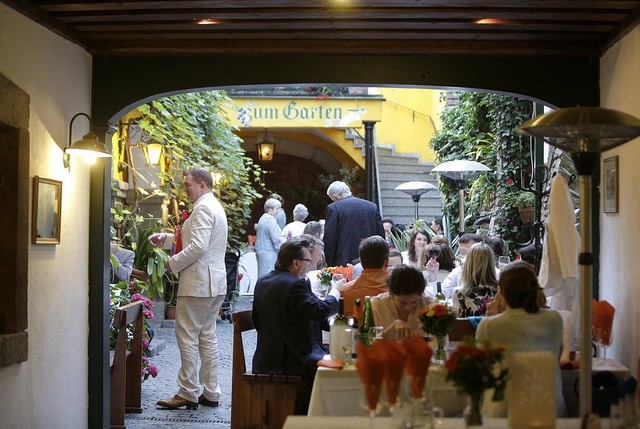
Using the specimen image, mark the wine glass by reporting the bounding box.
[498,256,510,270]
[359,384,383,429]
[591,327,601,363]
[332,274,343,283]
[405,374,432,404]
[597,328,614,366]
[380,381,401,413]
[429,245,440,259]
[342,345,352,365]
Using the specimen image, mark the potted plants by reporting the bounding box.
[514,190,535,225]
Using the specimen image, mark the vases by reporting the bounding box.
[462,387,487,426]
[432,333,447,363]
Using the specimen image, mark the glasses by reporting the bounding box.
[296,258,312,264]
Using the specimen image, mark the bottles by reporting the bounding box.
[359,295,376,347]
[334,297,348,325]
[435,281,445,300]
[351,298,363,358]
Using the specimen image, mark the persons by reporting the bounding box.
[401,229,432,272]
[430,216,444,235]
[252,238,346,376]
[268,193,286,231]
[339,235,393,317]
[388,250,403,269]
[382,219,395,238]
[254,198,284,281]
[289,234,329,355]
[322,178,386,268]
[485,259,551,316]
[430,235,456,273]
[280,203,309,243]
[364,263,435,342]
[148,167,228,410]
[474,267,570,418]
[304,220,323,238]
[486,235,504,269]
[425,233,500,301]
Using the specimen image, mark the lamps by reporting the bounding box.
[140,131,165,169]
[67,131,114,167]
[253,138,275,163]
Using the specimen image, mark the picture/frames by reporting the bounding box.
[31,174,63,245]
[601,155,620,214]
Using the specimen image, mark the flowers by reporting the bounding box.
[415,305,457,336]
[443,345,509,397]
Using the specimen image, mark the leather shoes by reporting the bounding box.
[197,394,218,407]
[156,394,197,409]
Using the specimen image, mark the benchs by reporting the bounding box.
[111,299,144,429]
[228,307,306,428]
